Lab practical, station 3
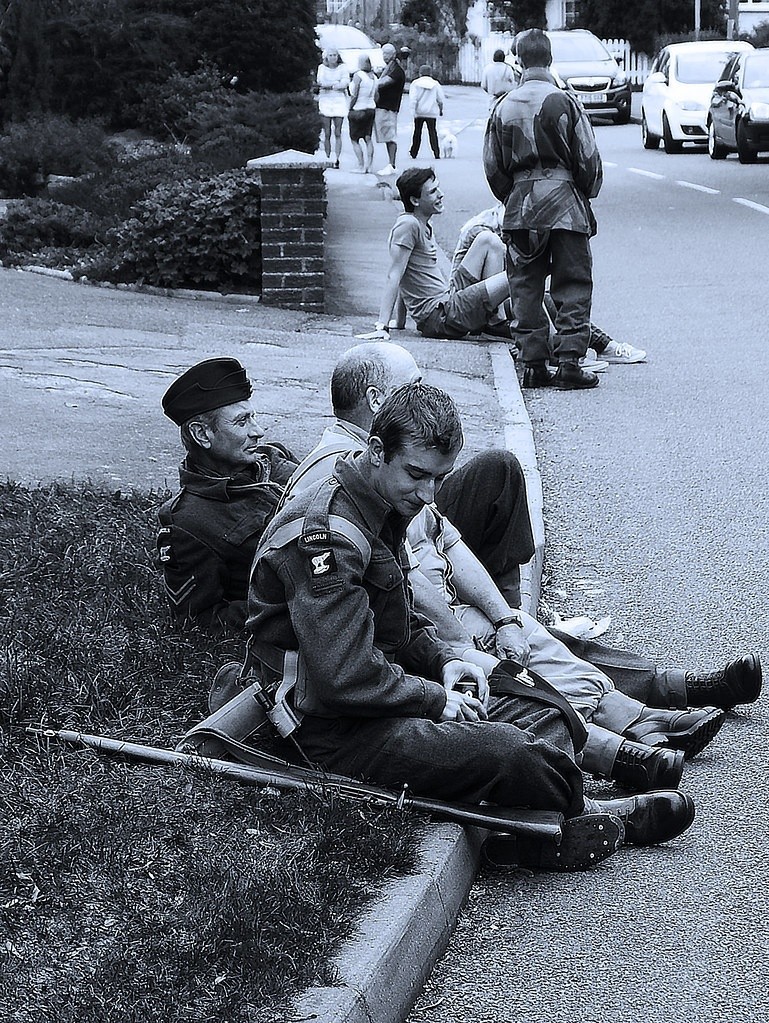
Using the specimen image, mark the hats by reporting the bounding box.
[162,357,251,426]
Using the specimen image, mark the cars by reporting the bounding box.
[315,22,389,96]
[502,27,633,126]
[704,46,768,165]
[639,40,756,155]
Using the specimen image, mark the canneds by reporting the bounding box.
[453,681,478,699]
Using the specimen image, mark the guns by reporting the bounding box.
[6,718,564,843]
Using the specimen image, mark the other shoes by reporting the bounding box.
[351,167,371,174]
[326,159,339,169]
[377,165,397,177]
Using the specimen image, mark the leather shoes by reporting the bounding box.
[486,813,626,870]
[685,651,763,710]
[624,708,724,755]
[579,789,695,845]
[554,361,600,389]
[611,736,686,787]
[522,366,553,389]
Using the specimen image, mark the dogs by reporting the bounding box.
[438,128,458,159]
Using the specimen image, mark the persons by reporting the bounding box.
[410,64,445,159]
[315,48,350,170]
[482,50,515,111]
[481,27,603,391]
[152,341,762,873]
[353,167,646,371]
[375,43,406,175]
[347,55,380,173]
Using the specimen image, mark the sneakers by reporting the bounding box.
[481,321,517,344]
[578,357,610,373]
[594,340,645,364]
